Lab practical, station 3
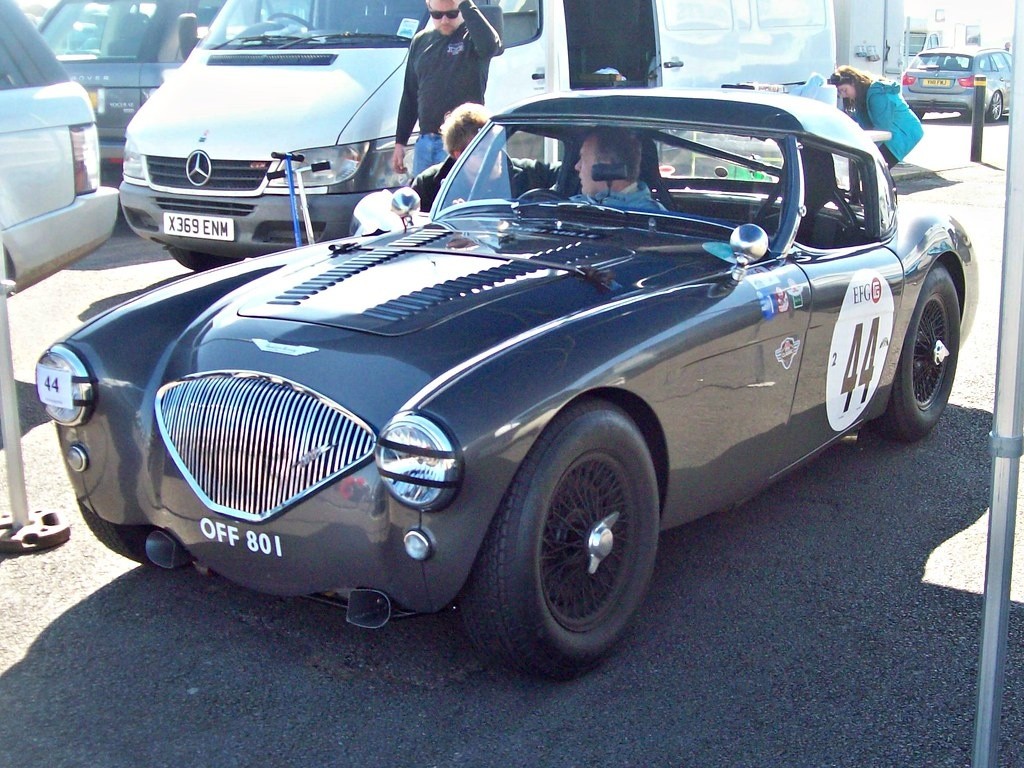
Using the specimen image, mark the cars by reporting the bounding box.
[901,45,1013,125]
[30,88,979,684]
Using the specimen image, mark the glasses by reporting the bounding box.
[830,73,851,85]
[429,9,460,19]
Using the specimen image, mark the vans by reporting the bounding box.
[903,28,943,70]
[0,0,120,302]
[35,0,335,188]
[117,0,840,277]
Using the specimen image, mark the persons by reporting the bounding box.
[566,128,668,214]
[826,65,925,204]
[413,101,580,214]
[389,0,505,180]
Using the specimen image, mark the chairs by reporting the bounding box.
[106,12,150,59]
[636,135,679,214]
[944,58,963,71]
[752,142,862,250]
